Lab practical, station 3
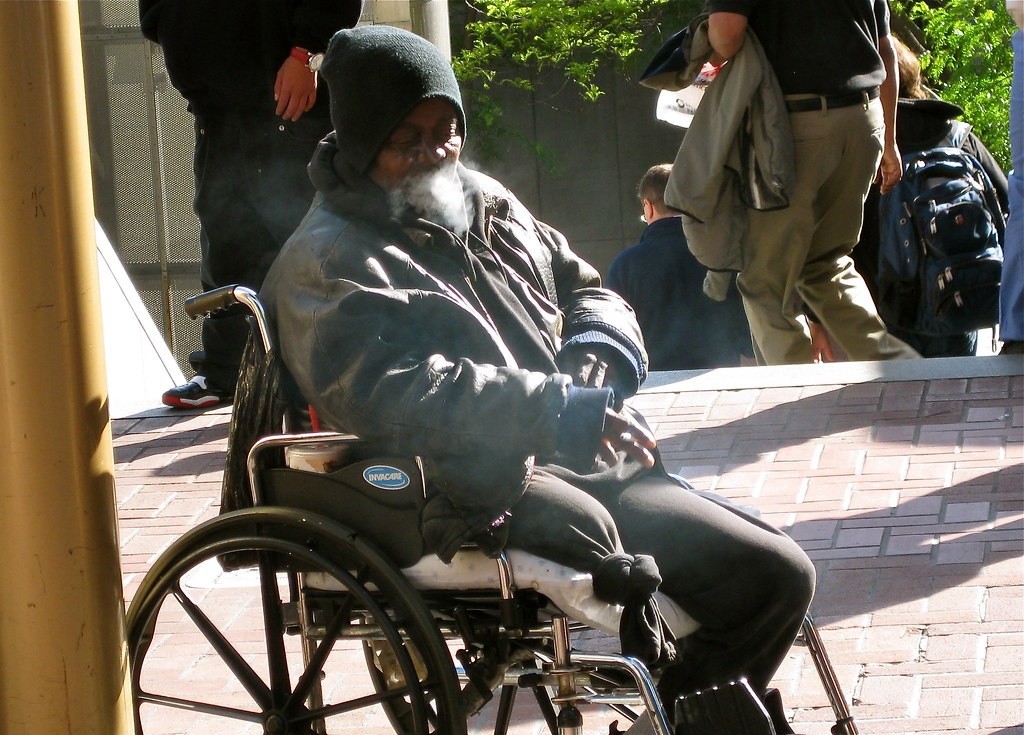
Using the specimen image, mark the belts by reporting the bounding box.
[784,85,881,113]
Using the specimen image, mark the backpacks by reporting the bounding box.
[867,117,1007,338]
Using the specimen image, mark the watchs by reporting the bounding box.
[292,49,324,74]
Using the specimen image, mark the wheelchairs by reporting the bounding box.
[119,276,862,735]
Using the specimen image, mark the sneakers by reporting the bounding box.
[162,371,235,410]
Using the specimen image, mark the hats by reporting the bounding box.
[320,25,467,176]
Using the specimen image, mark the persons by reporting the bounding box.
[609,161,756,371]
[707,0,1023,366]
[263,23,815,732]
[137,0,364,408]
